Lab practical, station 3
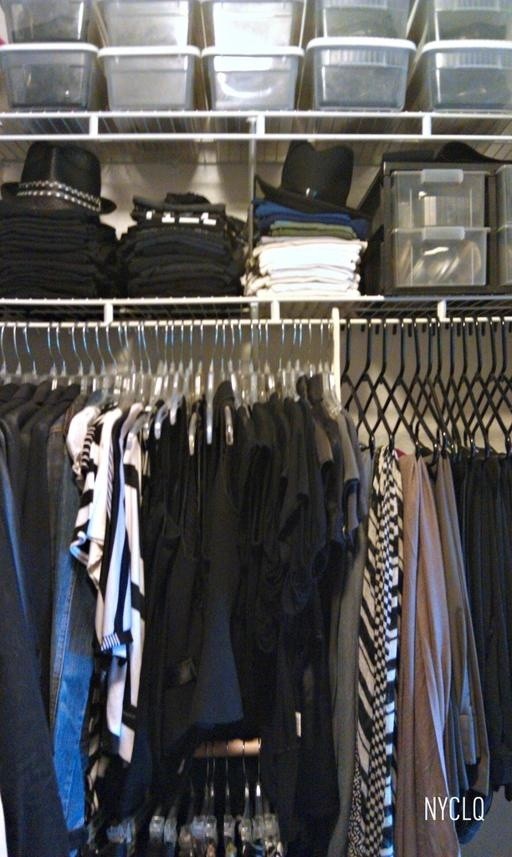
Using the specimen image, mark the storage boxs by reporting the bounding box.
[494,222,511,287]
[1,0,511,112]
[494,162,511,227]
[392,168,490,227]
[391,229,490,286]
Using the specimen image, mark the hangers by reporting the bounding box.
[1,314,510,463]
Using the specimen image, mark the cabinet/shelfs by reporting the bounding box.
[1,103,512,329]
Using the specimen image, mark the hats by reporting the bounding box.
[255,140,373,218]
[0,140,117,218]
